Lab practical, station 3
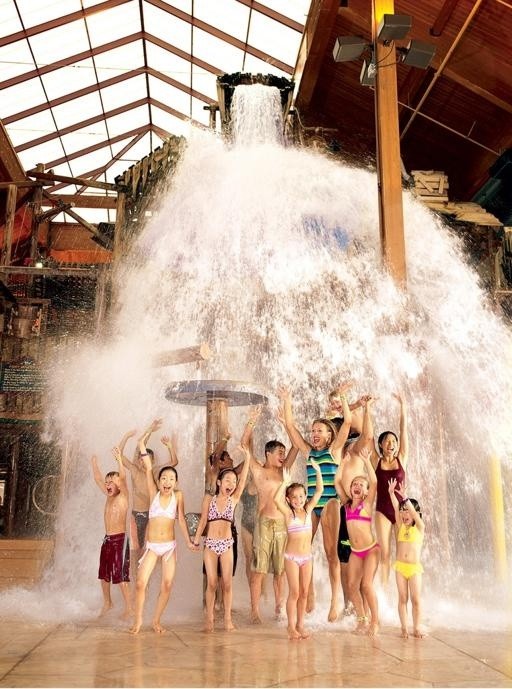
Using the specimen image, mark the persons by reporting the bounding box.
[387,478,427,639]
[204,386,409,624]
[128,435,202,634]
[334,447,381,636]
[92,446,131,615]
[191,445,250,635]
[273,458,324,639]
[120,420,179,555]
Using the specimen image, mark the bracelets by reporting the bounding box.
[192,541,200,546]
